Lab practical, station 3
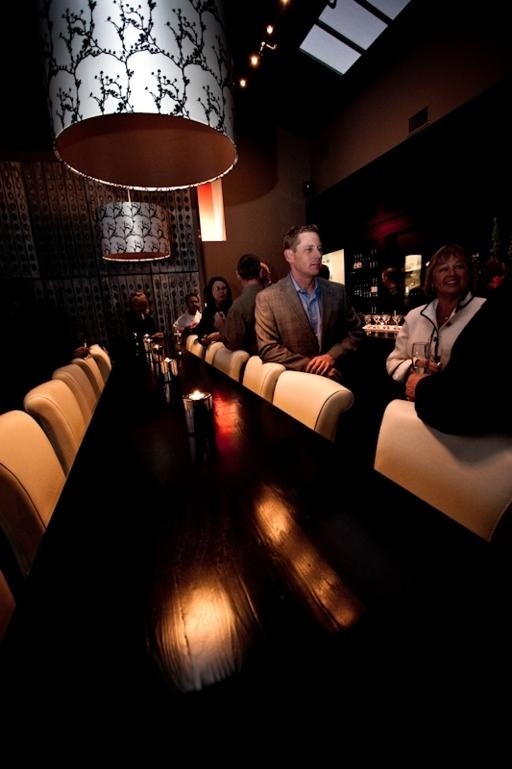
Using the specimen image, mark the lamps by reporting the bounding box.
[96,187,173,263]
[46,0,239,194]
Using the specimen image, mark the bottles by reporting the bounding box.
[352,249,378,316]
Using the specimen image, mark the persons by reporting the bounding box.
[127,224,512,437]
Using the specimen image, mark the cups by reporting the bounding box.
[180,392,214,435]
[411,343,430,374]
[133,332,179,382]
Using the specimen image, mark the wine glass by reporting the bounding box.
[364,314,402,331]
